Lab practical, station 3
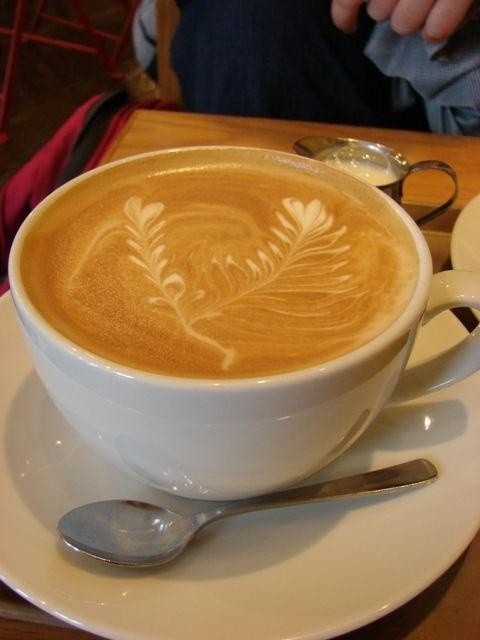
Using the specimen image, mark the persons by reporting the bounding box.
[131,0,480,137]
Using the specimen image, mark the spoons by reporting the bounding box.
[54,458,443,567]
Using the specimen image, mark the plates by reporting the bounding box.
[0,288,479,640]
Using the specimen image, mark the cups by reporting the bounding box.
[290,130,461,228]
[7,145,479,501]
